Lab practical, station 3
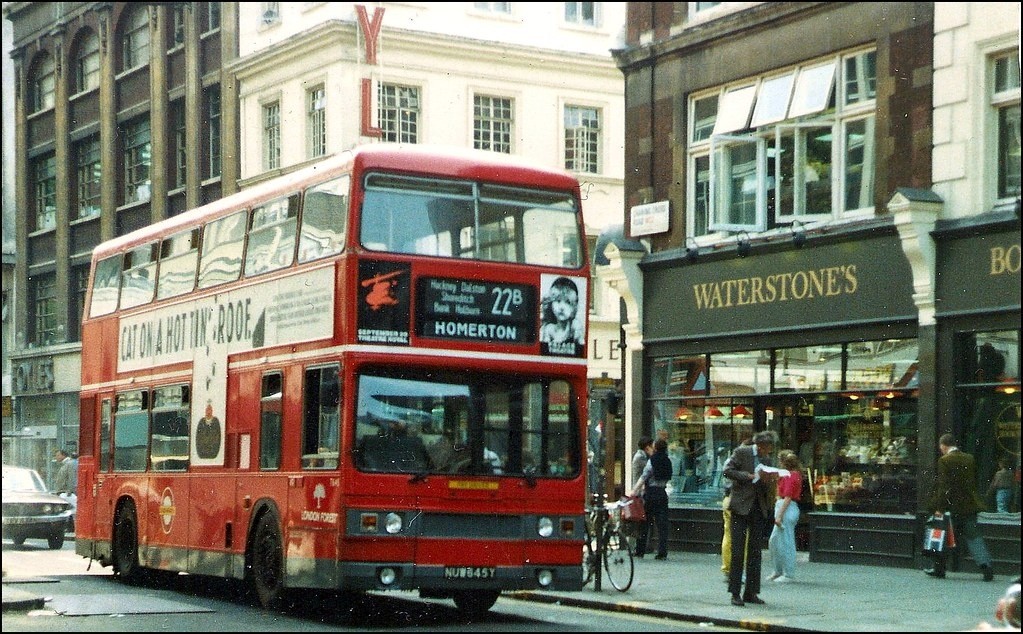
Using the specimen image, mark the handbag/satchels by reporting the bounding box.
[619,496,647,522]
[922,511,956,556]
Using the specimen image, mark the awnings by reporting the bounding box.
[711,64,836,138]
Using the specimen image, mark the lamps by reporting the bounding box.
[993,377,1021,394]
[672,406,694,420]
[877,391,904,398]
[729,405,753,418]
[842,393,865,401]
[700,406,724,418]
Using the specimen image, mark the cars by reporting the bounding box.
[2,464,71,549]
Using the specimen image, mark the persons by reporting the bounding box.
[721,431,780,606]
[542,276,585,345]
[767,451,802,583]
[55,449,78,532]
[923,434,994,582]
[631,438,673,559]
[371,417,570,475]
[986,459,1013,513]
[588,451,598,500]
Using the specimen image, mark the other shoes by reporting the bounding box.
[629,550,645,558]
[742,592,764,604]
[655,553,666,561]
[983,569,993,582]
[767,572,782,581]
[731,595,746,606]
[924,567,946,578]
[773,575,795,584]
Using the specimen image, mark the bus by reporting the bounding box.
[74,140,587,610]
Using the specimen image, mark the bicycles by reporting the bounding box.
[584,494,635,592]
